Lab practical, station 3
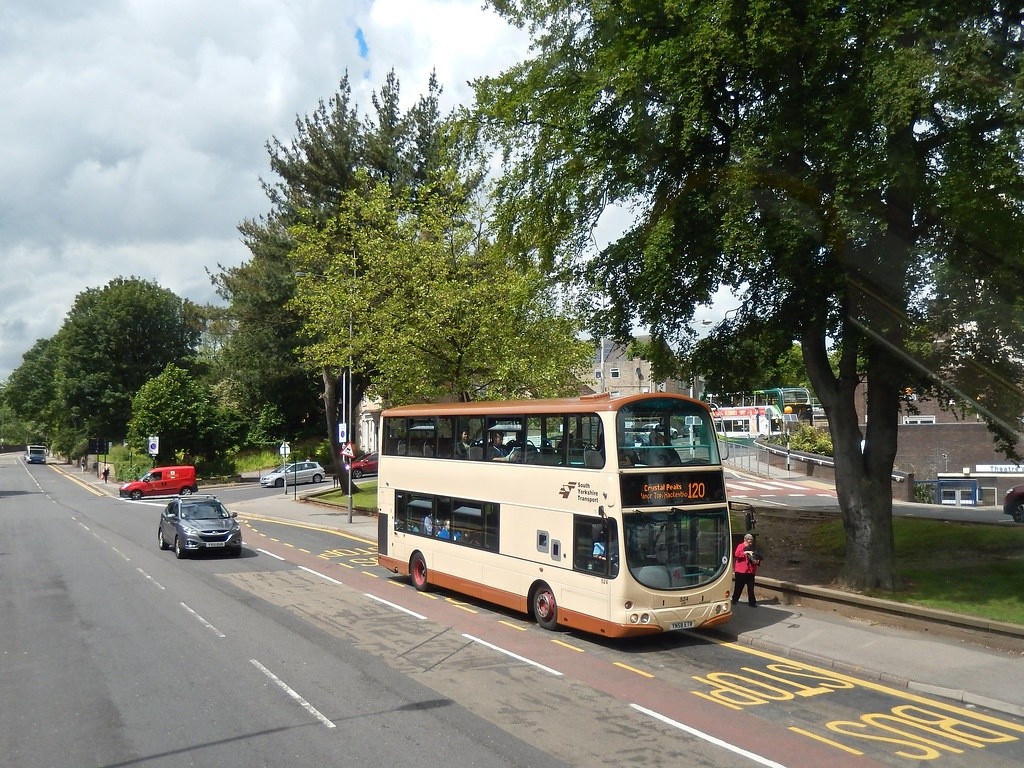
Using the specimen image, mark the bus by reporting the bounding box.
[376,389,757,640]
[24,444,47,465]
[698,387,815,446]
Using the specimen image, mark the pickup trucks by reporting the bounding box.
[118,465,198,500]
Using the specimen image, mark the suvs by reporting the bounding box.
[158,494,242,559]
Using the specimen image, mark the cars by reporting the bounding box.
[259,460,326,489]
[344,450,379,479]
[1003,483,1024,524]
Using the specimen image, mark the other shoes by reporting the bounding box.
[732,599,737,604]
[749,603,757,607]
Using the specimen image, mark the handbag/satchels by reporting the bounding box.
[101,474,104,480]
[743,544,763,562]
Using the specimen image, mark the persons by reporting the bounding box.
[150,474,161,481]
[489,432,520,462]
[456,427,475,457]
[559,431,673,467]
[593,529,619,565]
[102,465,110,484]
[423,509,462,542]
[80,456,87,473]
[732,533,760,607]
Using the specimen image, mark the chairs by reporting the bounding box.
[398,435,605,470]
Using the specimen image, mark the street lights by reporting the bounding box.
[295,271,353,523]
